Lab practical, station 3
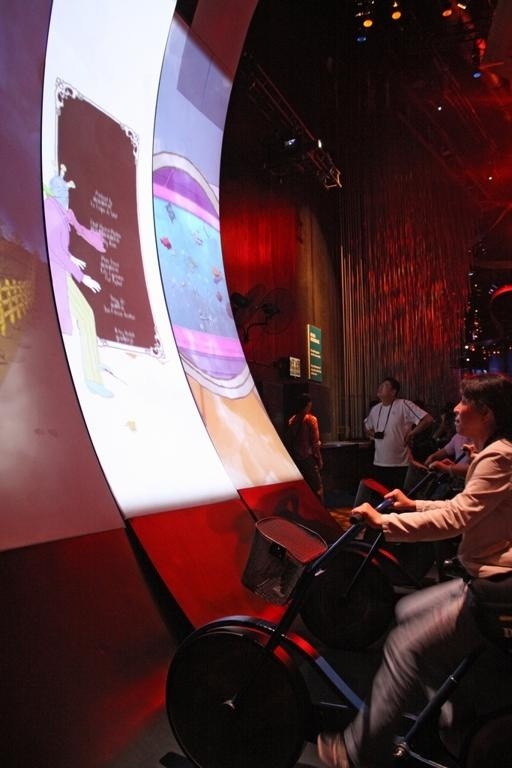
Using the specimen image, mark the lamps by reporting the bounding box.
[284,135,296,147]
[356,0,402,28]
[314,139,322,149]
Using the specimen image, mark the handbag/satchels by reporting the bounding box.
[453,576,512,656]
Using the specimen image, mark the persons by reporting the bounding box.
[288,394,325,507]
[429,442,475,477]
[314,371,510,767]
[364,377,436,505]
[423,432,476,482]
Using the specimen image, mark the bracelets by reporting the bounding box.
[447,462,453,475]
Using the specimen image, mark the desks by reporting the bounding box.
[321,441,372,497]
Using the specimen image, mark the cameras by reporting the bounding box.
[374,432,383,439]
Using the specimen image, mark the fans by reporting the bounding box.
[230,285,295,343]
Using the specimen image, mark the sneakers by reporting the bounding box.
[318,730,350,768]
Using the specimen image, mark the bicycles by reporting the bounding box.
[164,432,512,768]
